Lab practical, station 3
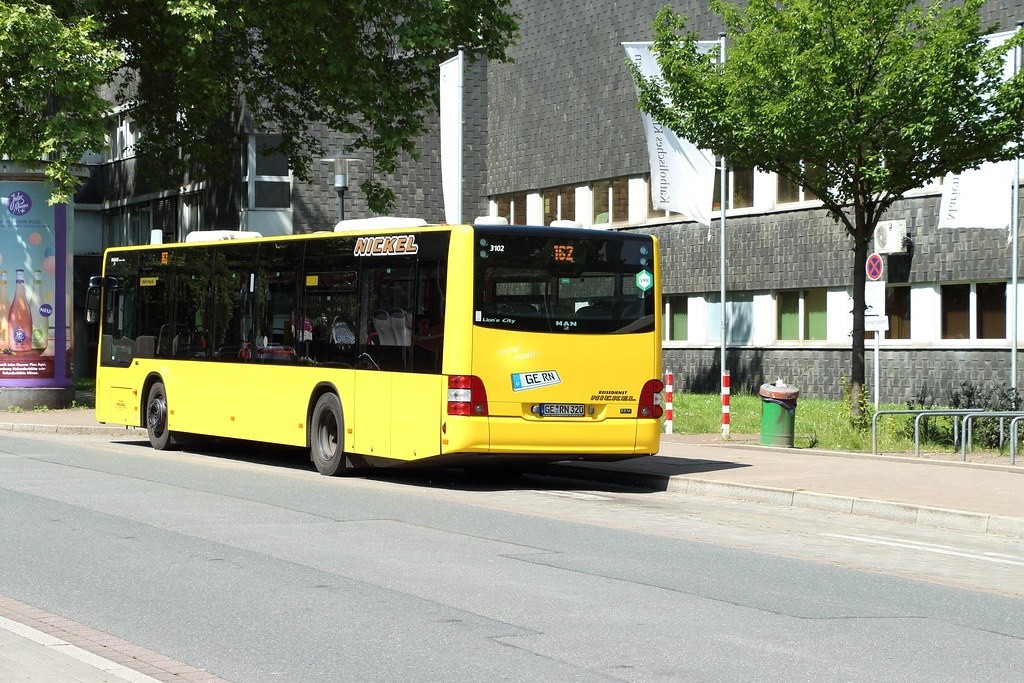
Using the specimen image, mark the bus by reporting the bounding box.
[84,217,666,477]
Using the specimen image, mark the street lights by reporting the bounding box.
[319,158,366,222]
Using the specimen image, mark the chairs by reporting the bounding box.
[156,307,443,374]
[486,297,641,320]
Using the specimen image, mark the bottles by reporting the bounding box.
[27,270,48,349]
[0,269,13,355]
[7,269,32,352]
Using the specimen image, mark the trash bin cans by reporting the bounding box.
[759,380,799,447]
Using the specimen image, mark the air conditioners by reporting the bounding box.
[875,220,910,255]
